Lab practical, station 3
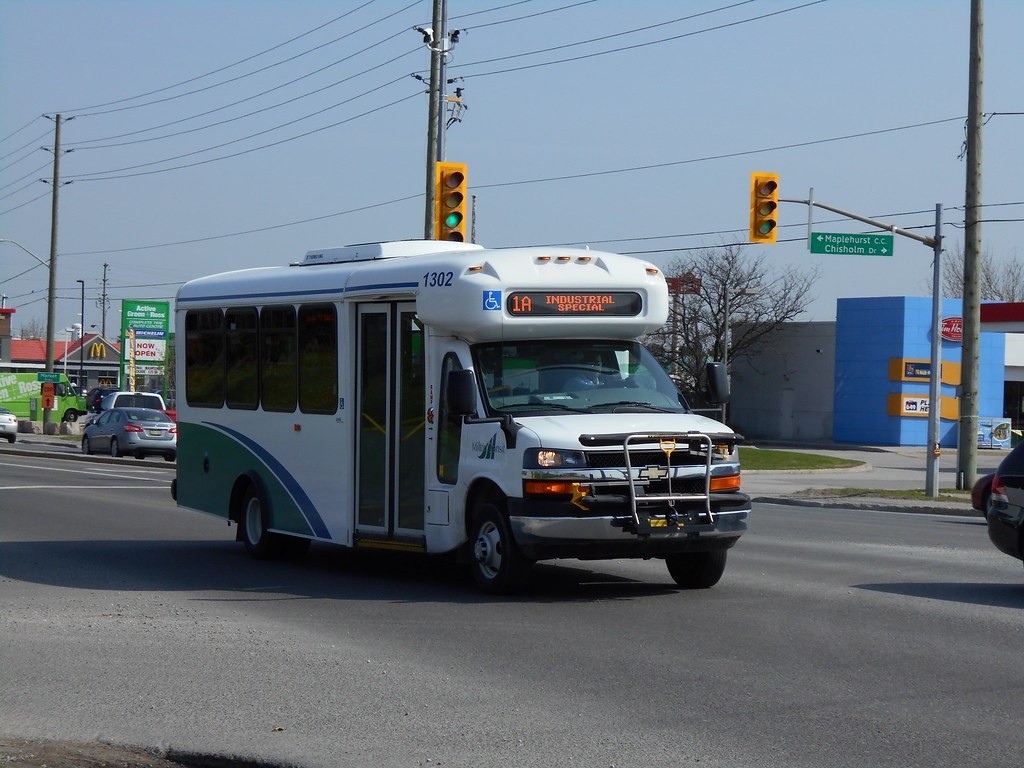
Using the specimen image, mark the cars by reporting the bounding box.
[0,407,18,443]
[987,442,1024,562]
[153,389,176,407]
[85,387,120,413]
[972,472,994,519]
[72,386,89,397]
[84,392,166,430]
[82,407,177,462]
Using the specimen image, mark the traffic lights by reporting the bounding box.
[436,162,467,243]
[749,171,779,244]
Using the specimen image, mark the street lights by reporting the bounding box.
[76,280,85,397]
[0,238,58,435]
[77,313,84,337]
[91,324,105,338]
[722,288,759,424]
[64,328,75,375]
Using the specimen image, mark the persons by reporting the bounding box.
[561,352,637,393]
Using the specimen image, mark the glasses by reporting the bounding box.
[586,362,602,368]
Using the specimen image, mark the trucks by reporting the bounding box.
[0,373,87,422]
[171,239,753,588]
